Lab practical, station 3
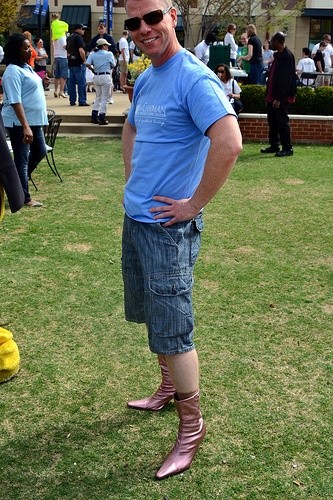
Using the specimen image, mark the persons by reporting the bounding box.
[237,33,250,75]
[87,25,118,104]
[196,32,215,63]
[50,13,69,98]
[66,23,90,106]
[214,63,241,110]
[313,42,327,86]
[262,40,274,70]
[23,31,48,77]
[260,32,295,157]
[311,34,333,86]
[116,30,129,90]
[237,24,264,84]
[224,24,238,66]
[1,34,49,206]
[86,38,115,125]
[121,0,244,479]
[130,40,142,61]
[294,47,316,87]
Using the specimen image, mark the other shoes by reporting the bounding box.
[79,102,90,107]
[25,200,42,207]
[70,102,76,106]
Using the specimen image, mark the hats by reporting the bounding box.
[73,23,88,29]
[96,38,112,46]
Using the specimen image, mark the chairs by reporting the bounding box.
[300,72,318,87]
[28,110,64,190]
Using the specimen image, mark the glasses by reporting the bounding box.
[124,6,172,31]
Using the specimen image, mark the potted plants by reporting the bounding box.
[123,53,152,102]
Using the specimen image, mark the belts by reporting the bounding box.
[95,72,110,74]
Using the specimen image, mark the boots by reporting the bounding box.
[127,357,175,412]
[155,391,206,480]
[91,110,99,124]
[98,113,108,125]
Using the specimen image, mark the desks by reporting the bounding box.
[230,69,248,82]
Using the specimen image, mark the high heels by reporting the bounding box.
[58,92,68,98]
[87,88,90,92]
[54,93,57,98]
[92,87,96,92]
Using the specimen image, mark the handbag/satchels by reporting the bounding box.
[133,46,141,57]
[115,42,121,55]
[231,81,244,114]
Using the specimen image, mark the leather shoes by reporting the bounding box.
[260,145,280,153]
[274,149,293,157]
